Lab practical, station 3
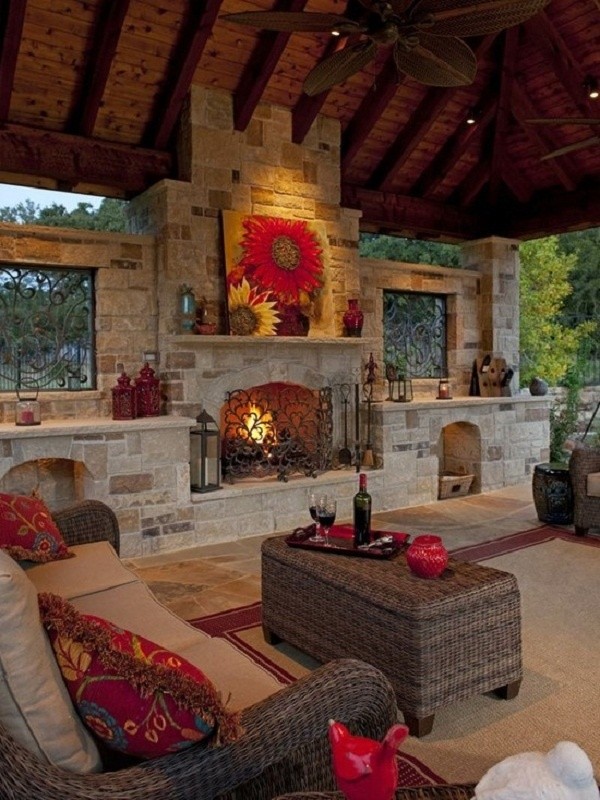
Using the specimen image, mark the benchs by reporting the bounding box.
[260,530,526,739]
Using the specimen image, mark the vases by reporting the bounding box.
[343,299,364,338]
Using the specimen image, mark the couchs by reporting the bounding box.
[0,500,398,800]
[570,442,600,536]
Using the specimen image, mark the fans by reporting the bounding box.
[219,0,557,96]
[524,115,600,161]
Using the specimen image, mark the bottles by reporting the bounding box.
[112,372,135,419]
[343,299,364,338]
[353,473,372,548]
[135,361,159,417]
[15,398,41,427]
[404,534,449,580]
[439,380,449,397]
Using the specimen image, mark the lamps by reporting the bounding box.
[188,408,221,494]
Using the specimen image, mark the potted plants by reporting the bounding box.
[530,335,595,524]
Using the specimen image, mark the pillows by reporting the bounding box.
[0,492,75,561]
[37,590,247,761]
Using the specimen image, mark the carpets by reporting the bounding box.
[189,527,600,800]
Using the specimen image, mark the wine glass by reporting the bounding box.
[316,498,337,548]
[309,494,327,543]
